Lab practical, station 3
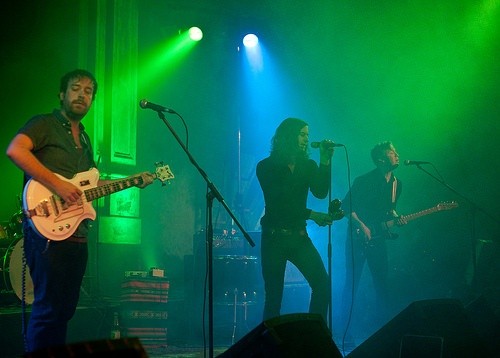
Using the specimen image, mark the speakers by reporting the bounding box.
[216,311,344,358]
[347,296,482,358]
[21,338,151,358]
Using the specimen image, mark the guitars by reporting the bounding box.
[355,201,458,250]
[22,164,175,242]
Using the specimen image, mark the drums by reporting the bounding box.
[191,233,243,272]
[8,210,24,236]
[0,235,34,307]
[209,254,260,305]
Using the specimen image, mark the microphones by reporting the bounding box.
[139,99,174,114]
[404,160,430,164]
[310,142,344,148]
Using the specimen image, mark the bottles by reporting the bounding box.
[111,312,120,340]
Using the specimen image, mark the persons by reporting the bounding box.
[342,143,408,351]
[256,118,334,326]
[6,70,153,354]
